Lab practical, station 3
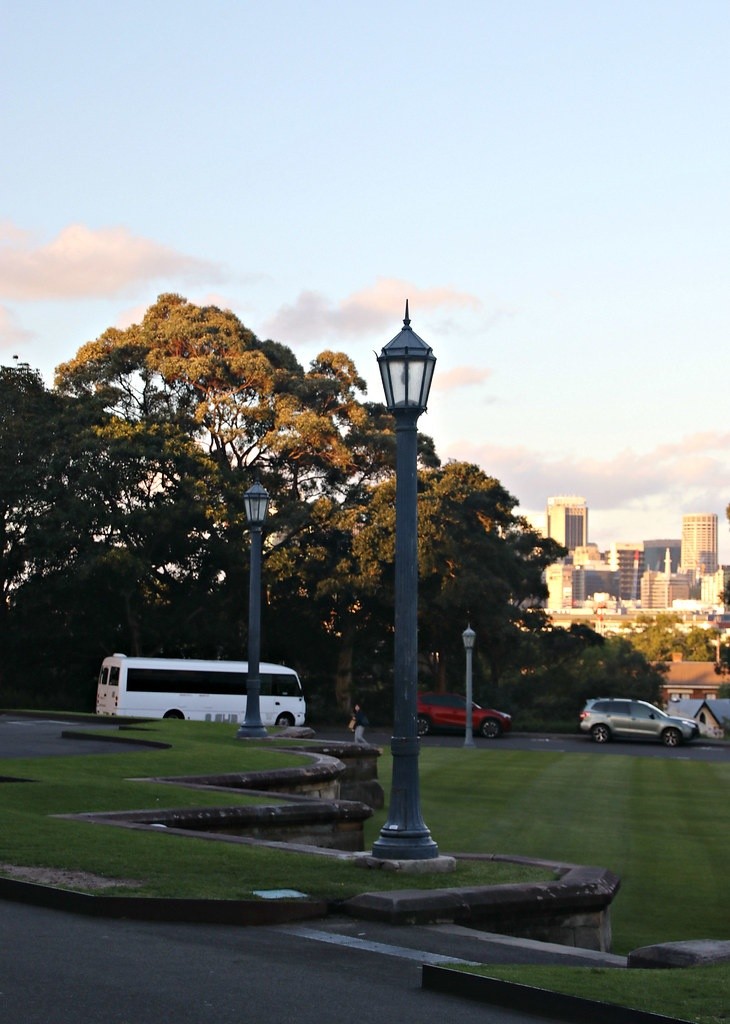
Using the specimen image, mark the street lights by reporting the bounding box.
[242,475,270,735]
[463,624,476,749]
[370,296,442,859]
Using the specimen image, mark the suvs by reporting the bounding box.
[415,691,512,738]
[579,698,698,747]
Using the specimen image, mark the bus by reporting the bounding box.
[95,653,306,727]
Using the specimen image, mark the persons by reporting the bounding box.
[351,704,369,744]
